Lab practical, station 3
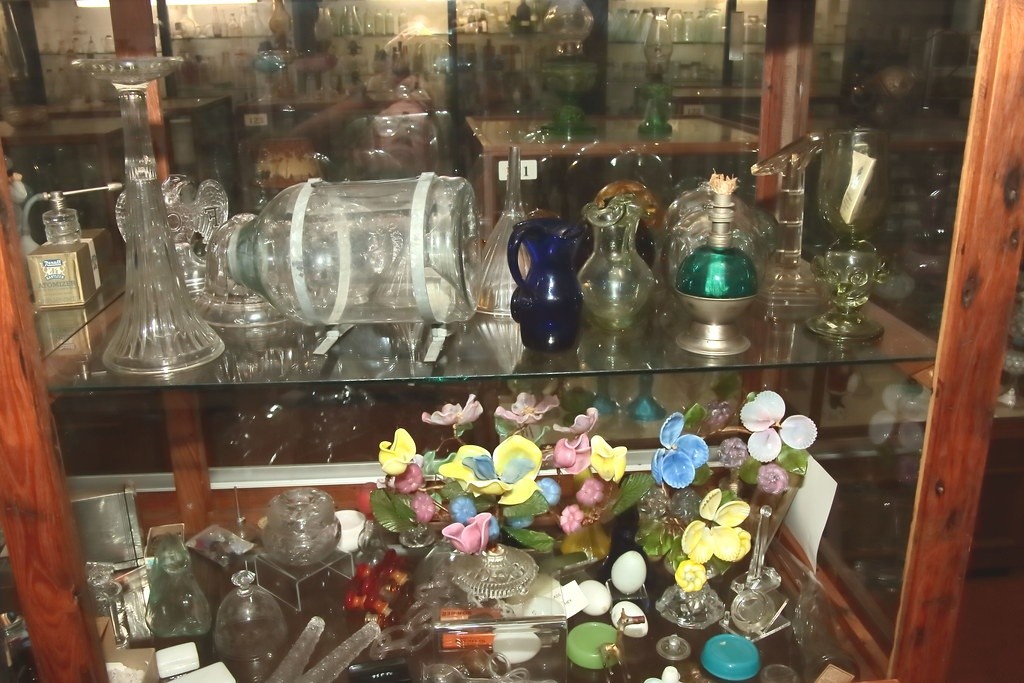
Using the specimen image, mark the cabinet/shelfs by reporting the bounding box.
[9,0,858,215]
[0,0,1024,683]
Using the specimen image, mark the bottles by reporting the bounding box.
[170,4,849,101]
[208,174,483,327]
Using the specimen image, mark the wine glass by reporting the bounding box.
[473,118,533,318]
[71,55,225,375]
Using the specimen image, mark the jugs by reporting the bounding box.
[507,218,589,351]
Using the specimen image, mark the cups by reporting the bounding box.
[119,177,229,298]
